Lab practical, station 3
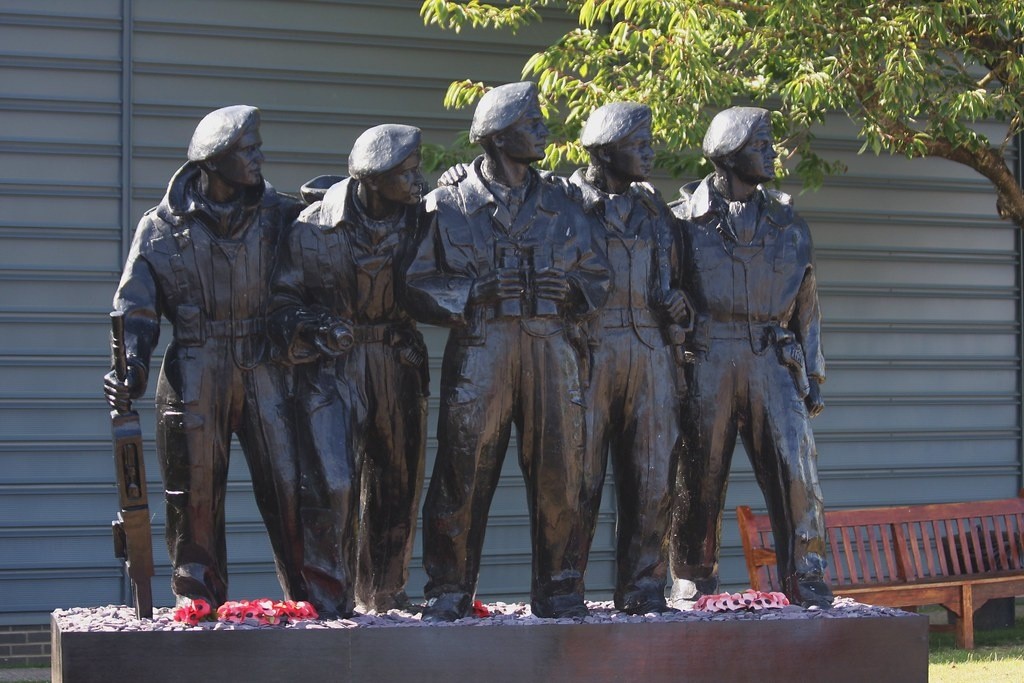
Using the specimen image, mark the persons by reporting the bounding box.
[104,82,835,622]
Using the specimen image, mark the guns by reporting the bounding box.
[109,311,155,620]
[387,322,432,398]
[765,325,812,397]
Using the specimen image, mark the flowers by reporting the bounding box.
[174,593,318,626]
[693,587,790,614]
[472,600,491,618]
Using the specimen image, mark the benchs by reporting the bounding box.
[734,486,1024,654]
